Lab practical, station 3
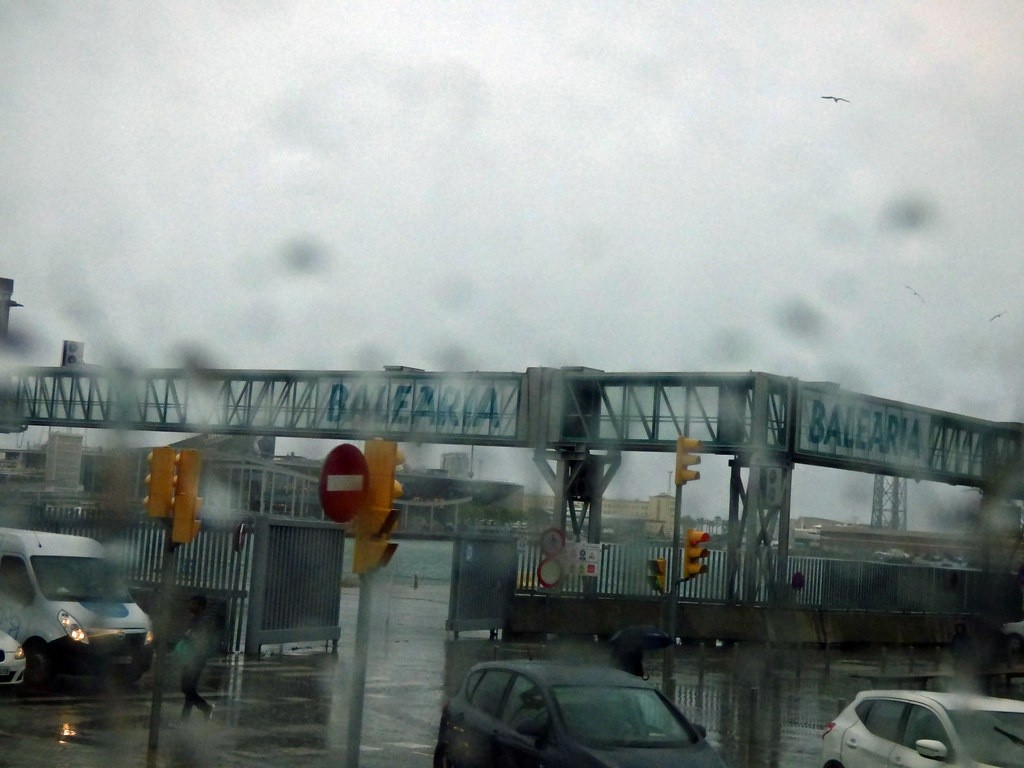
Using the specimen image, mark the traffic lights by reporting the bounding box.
[675,436,700,487]
[143,445,176,519]
[363,438,407,507]
[353,507,400,576]
[684,527,709,577]
[647,558,667,593]
[172,448,207,544]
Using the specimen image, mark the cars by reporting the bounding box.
[0,631,26,687]
[784,526,1024,578]
[435,660,728,767]
[816,689,1024,768]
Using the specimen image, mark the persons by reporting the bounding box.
[167,591,215,728]
[615,637,644,678]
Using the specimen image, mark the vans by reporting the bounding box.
[0,526,154,686]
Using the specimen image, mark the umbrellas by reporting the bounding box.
[608,625,670,680]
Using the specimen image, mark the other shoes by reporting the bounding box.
[167,722,189,731]
[203,702,215,724]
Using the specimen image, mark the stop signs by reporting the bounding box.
[321,444,370,521]
